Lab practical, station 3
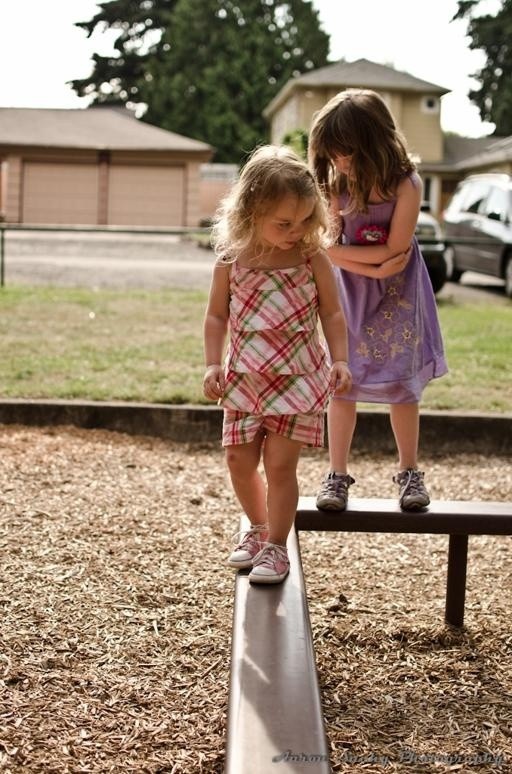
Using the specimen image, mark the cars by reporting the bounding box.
[414,171,512,299]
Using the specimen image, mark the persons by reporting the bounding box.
[305,84,447,514]
[199,141,356,588]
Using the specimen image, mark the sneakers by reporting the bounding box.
[316,472,355,510]
[392,468,430,509]
[228,521,269,567]
[249,542,290,583]
